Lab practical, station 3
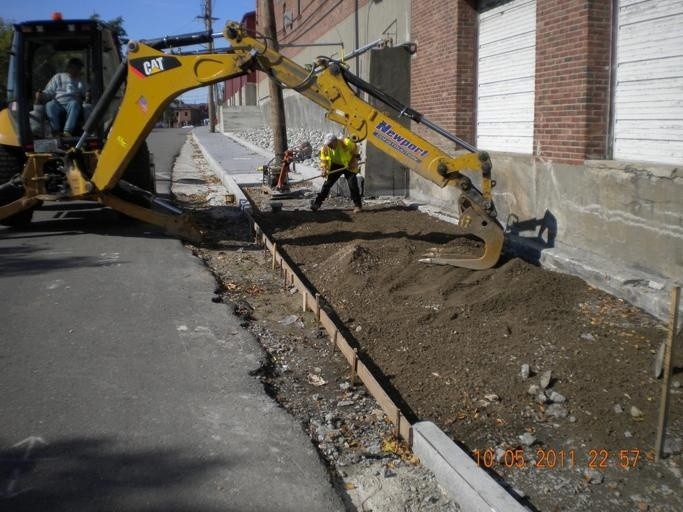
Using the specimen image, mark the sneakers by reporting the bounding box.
[310,205,319,211]
[353,206,363,213]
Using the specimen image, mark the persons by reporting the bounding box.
[311,134,362,214]
[35,57,94,138]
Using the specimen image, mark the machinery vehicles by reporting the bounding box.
[0,11,505,272]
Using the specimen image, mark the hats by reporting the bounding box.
[323,134,337,145]
